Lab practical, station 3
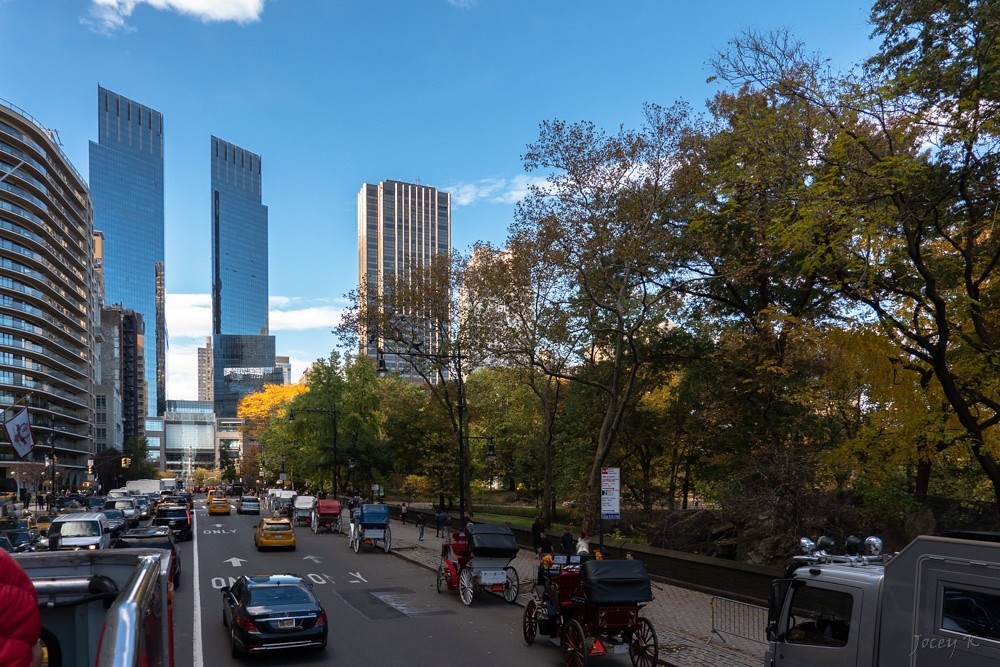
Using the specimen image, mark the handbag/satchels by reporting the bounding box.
[541,532,546,538]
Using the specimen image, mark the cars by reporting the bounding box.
[0,478,195,553]
[220,573,328,661]
[253,510,296,552]
[191,482,243,506]
[209,497,231,516]
[236,496,261,515]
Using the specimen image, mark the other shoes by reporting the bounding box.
[538,548,542,553]
[535,555,538,559]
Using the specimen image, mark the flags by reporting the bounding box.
[3,406,36,457]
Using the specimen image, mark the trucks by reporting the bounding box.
[763,534,1000,667]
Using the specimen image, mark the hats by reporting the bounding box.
[565,527,569,530]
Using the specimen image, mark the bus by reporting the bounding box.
[9,547,173,667]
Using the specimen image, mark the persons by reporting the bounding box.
[559,527,575,556]
[10,491,53,511]
[694,497,704,504]
[532,516,554,560]
[399,502,408,525]
[414,512,427,541]
[576,531,635,560]
[434,510,452,540]
[350,496,371,509]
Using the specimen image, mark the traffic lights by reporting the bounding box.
[88,465,93,475]
[126,457,131,465]
[53,455,58,465]
[122,458,127,468]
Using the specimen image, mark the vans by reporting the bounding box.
[39,513,112,550]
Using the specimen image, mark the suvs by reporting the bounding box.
[113,525,181,591]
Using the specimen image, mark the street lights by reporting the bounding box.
[375,350,497,530]
[289,407,357,500]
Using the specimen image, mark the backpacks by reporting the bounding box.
[402,506,406,513]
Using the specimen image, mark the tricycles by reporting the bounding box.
[265,489,342,535]
[437,525,519,607]
[350,503,392,554]
[523,554,659,667]
